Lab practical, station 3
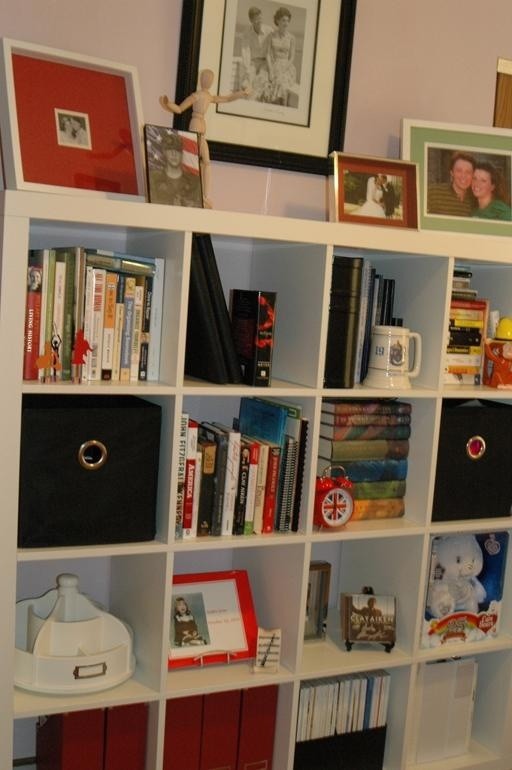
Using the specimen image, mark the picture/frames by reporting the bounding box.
[327,150,421,228]
[171,0,359,178]
[168,569,258,671]
[2,36,147,198]
[397,115,512,239]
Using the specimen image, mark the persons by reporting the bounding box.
[158,69,251,209]
[425,152,512,222]
[59,115,89,146]
[148,134,203,208]
[240,6,297,105]
[348,172,397,219]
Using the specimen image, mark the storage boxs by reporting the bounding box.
[19,392,164,549]
[431,399,511,522]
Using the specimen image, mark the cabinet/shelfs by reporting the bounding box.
[0,187,512,770]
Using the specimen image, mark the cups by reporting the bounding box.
[361,325,424,390]
